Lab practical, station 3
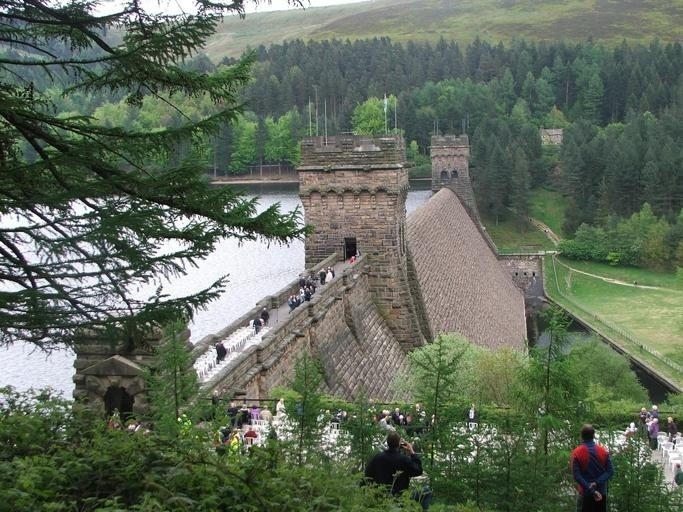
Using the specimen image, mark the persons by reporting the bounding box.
[365,431,422,496]
[570,424,615,511]
[253,307,269,336]
[288,266,334,309]
[108,391,293,456]
[294,390,441,429]
[624,404,676,450]
[350,249,360,264]
[674,463,683,487]
[215,339,227,364]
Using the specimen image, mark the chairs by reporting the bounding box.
[236,409,683,512]
[193,319,272,379]
